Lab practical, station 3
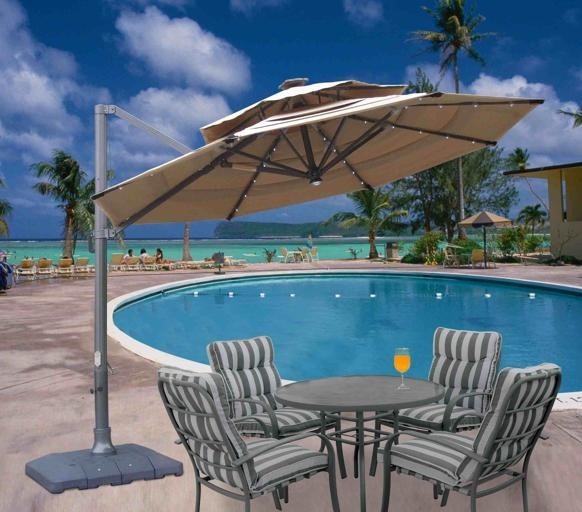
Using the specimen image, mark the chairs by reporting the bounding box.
[276,247,319,264]
[0,246,247,285]
[424,247,499,270]
[155,367,342,511]
[377,363,563,512]
[368,323,502,499]
[202,335,350,503]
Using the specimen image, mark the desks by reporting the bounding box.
[270,372,441,511]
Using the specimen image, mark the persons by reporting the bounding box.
[154,248,163,262]
[123,248,135,259]
[140,249,148,256]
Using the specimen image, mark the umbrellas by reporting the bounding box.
[91,78,548,455]
[457,208,511,267]
